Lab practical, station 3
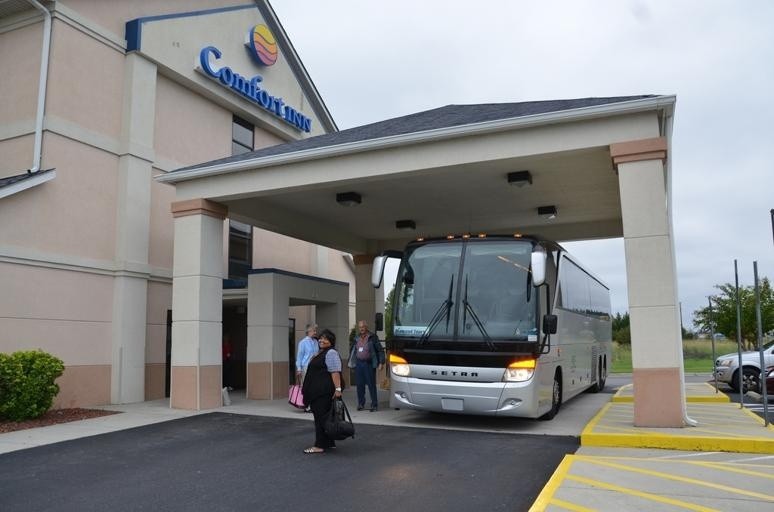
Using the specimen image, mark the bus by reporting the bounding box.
[367,231,613,426]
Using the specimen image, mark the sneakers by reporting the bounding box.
[303,447,324,453]
[371,405,376,411]
[357,404,363,410]
[324,443,335,448]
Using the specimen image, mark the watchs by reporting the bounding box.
[335,386,342,392]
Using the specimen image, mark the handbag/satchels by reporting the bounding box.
[322,396,354,440]
[288,385,304,407]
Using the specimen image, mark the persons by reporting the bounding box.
[223,331,234,391]
[345,319,385,412]
[295,323,320,413]
[301,329,343,455]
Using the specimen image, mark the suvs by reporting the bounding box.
[710,339,774,395]
